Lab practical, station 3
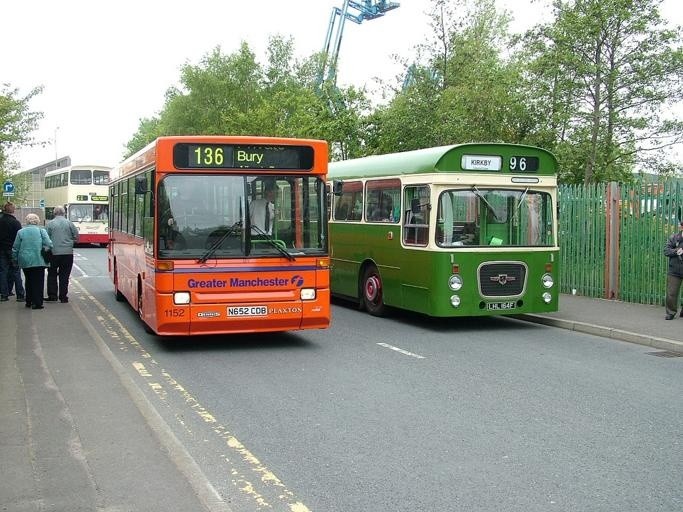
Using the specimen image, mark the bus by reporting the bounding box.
[39,165,109,246]
[105,135,332,338]
[250,141,563,322]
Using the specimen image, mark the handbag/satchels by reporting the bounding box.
[41,247,54,263]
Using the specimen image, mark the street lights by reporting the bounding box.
[54,126,61,169]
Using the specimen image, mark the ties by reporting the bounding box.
[263,202,271,233]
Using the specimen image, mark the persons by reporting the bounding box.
[663,220,683,321]
[0,201,25,301]
[97,208,108,219]
[166,184,201,245]
[12,213,53,309]
[0,203,15,296]
[249,183,279,250]
[44,205,78,303]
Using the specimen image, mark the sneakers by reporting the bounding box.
[32,305,45,309]
[9,293,15,296]
[26,303,32,307]
[0,297,9,301]
[16,298,26,302]
[43,297,58,302]
[60,298,69,303]
